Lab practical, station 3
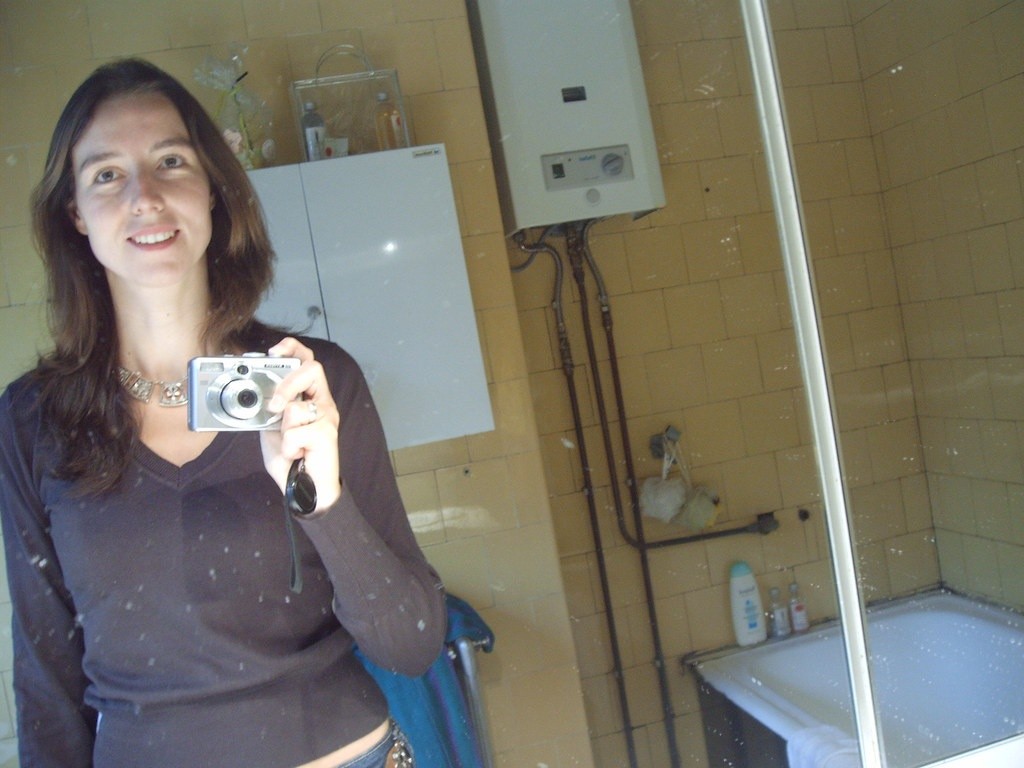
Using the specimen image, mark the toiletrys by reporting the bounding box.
[764,582,810,638]
[374,92,407,151]
[728,561,768,646]
[300,102,329,162]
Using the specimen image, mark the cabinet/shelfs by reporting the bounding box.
[245,142,496,451]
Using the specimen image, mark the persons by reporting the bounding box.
[0,57,449,768]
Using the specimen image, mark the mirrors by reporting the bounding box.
[0,0,864,768]
[761,0,1024,768]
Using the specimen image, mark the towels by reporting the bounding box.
[786,726,861,768]
[351,590,495,768]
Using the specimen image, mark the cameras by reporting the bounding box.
[187,352,301,434]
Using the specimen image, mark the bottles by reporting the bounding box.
[301,103,328,162]
[768,586,791,640]
[789,582,809,633]
[375,94,403,151]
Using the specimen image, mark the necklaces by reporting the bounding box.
[117,339,234,407]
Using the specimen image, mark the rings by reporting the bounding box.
[310,399,317,416]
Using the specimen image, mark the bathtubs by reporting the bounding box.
[685,585,1024,768]
